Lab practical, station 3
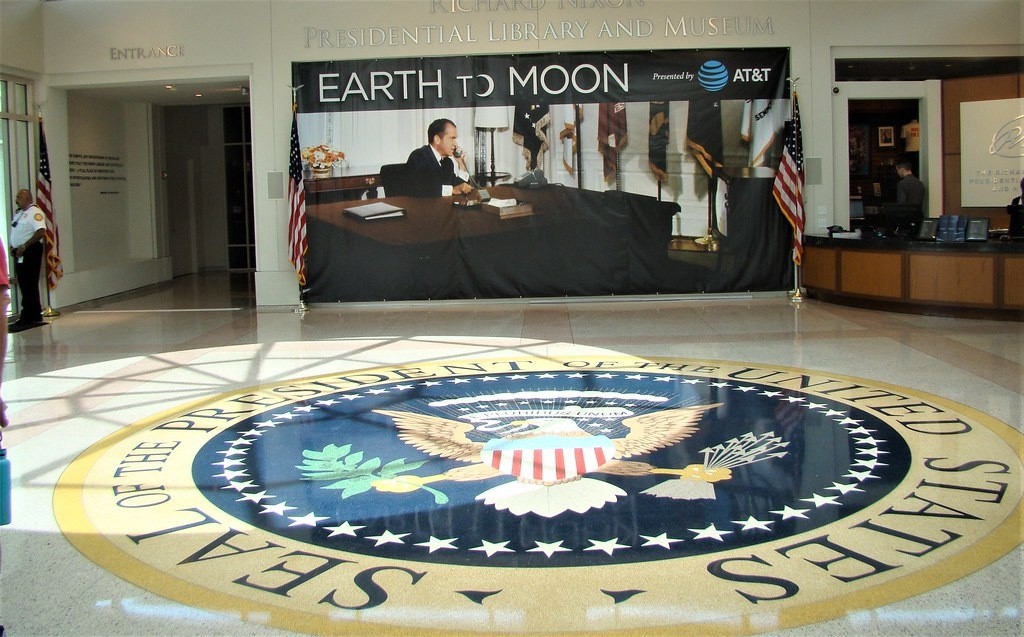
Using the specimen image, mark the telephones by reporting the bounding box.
[453,148,462,158]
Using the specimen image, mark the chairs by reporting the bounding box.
[380,164,406,197]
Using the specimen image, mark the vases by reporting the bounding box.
[312,167,331,179]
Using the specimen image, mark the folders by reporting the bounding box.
[343,202,406,222]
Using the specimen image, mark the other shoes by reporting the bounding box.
[14,315,43,326]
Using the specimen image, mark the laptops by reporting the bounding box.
[1008,205,1024,240]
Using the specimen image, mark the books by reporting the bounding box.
[343,201,408,223]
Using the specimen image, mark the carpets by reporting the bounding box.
[51,357,1024,637]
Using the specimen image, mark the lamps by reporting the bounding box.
[806,158,822,234]
[475,107,510,185]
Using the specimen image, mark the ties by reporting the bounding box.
[440,159,444,167]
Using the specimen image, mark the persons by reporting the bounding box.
[401,117,472,196]
[894,160,925,238]
[11,188,48,327]
[901,118,921,152]
[0,237,10,438]
[999,177,1024,240]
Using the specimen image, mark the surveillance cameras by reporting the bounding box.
[832,87,839,95]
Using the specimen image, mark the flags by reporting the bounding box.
[35,118,64,290]
[286,102,310,287]
[512,98,790,184]
[771,91,806,267]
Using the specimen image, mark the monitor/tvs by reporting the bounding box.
[880,203,922,239]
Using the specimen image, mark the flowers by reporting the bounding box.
[300,145,346,171]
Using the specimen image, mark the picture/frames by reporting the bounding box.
[879,127,894,147]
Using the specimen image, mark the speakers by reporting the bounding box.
[804,157,822,186]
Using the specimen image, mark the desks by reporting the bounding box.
[306,183,681,303]
[802,231,1024,324]
[305,173,382,205]
